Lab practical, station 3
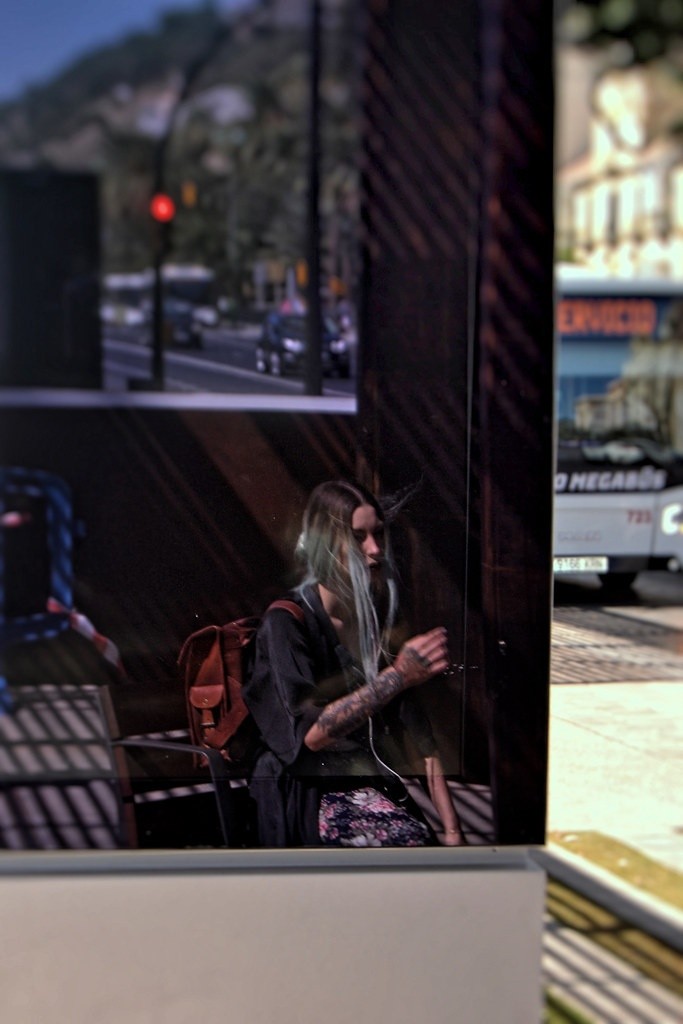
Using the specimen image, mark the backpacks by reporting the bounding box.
[173,601,311,772]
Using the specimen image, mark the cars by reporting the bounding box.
[256,313,351,381]
[147,302,202,347]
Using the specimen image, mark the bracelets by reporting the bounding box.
[444,829,462,835]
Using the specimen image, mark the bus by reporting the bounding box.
[553,262,682,590]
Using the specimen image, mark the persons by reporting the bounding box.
[242,478,470,849]
